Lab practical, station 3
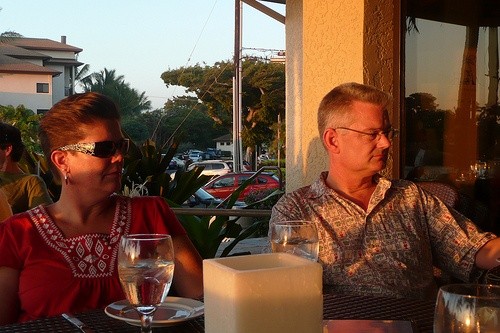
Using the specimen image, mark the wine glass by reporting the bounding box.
[117,233,175,333]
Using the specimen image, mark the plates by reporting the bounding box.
[105,296,205,327]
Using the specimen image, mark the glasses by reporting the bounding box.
[55,138,130,159]
[332,125,399,145]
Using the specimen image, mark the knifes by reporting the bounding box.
[61,312,92,333]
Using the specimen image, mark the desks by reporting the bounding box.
[0,295,438,333]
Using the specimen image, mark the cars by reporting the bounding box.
[161,148,284,200]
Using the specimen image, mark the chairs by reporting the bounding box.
[417,175,500,287]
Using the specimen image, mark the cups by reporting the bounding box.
[203,252,323,333]
[433,283,500,333]
[470,160,496,178]
[271,221,320,262]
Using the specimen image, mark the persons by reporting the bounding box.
[0,122,53,215]
[268,82,500,298]
[0,92,203,325]
